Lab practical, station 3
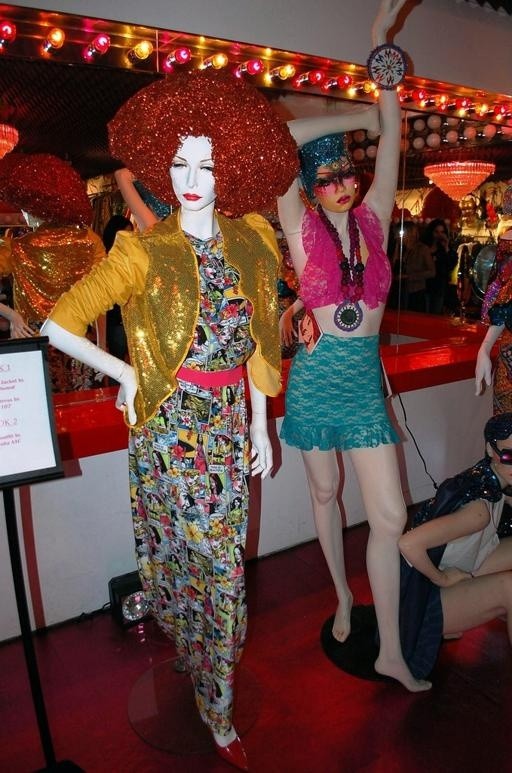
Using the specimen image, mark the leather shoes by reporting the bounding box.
[211,724,249,773]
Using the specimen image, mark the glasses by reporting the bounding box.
[488,439,511,465]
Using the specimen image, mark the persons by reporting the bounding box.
[101,215,134,387]
[397,412,511,648]
[276,263,306,360]
[0,152,108,394]
[389,218,457,315]
[39,64,282,773]
[114,166,158,233]
[474,275,512,416]
[276,1,435,693]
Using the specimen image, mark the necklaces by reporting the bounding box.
[317,204,365,333]
